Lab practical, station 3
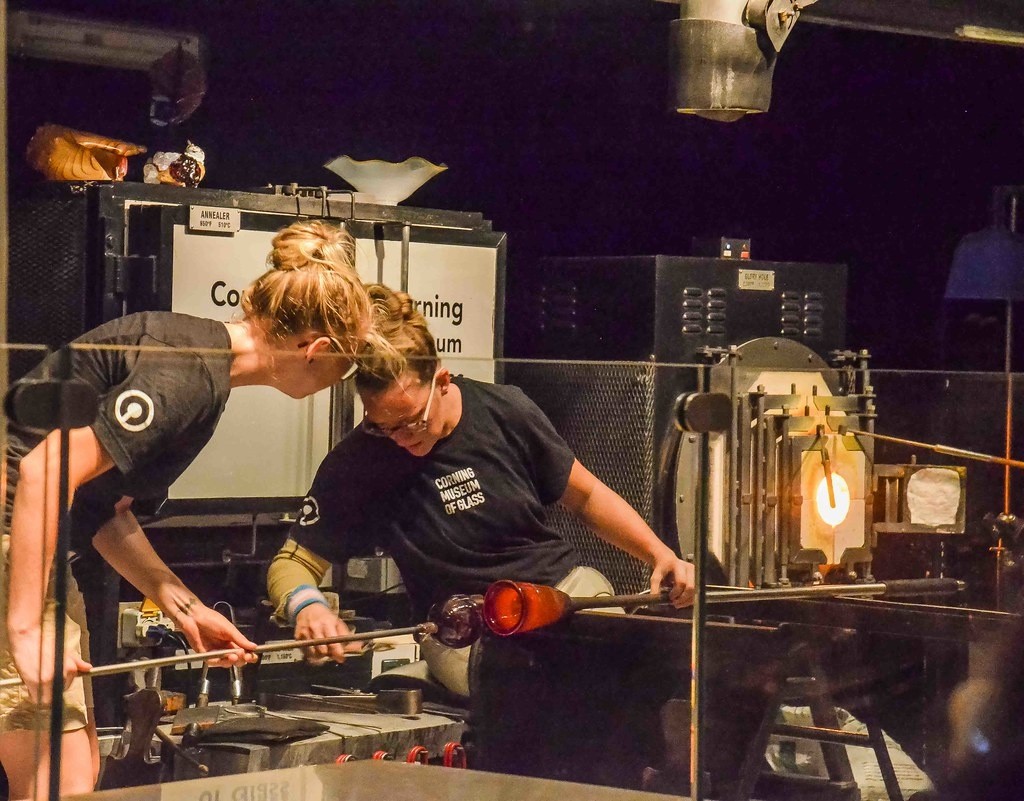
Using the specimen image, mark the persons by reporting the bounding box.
[1,219,373,800]
[267,284,694,697]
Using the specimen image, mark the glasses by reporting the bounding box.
[298,334,360,382]
[359,371,438,437]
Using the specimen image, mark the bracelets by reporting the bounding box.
[286,585,328,628]
